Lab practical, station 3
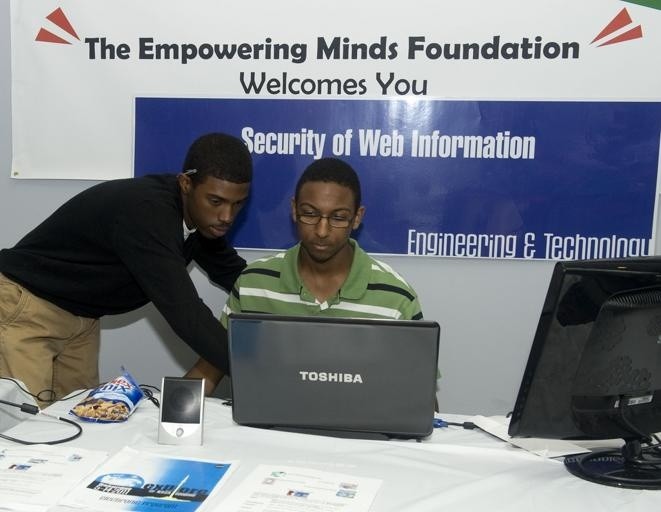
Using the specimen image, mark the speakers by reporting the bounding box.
[157,376,205,446]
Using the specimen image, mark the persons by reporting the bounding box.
[0,134,247,412]
[182,156,442,414]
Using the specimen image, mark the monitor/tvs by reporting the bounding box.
[507,254,661,490]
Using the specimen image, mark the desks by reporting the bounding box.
[0,385,661,512]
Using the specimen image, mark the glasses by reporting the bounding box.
[296,213,355,228]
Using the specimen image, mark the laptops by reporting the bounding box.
[225,311,441,441]
